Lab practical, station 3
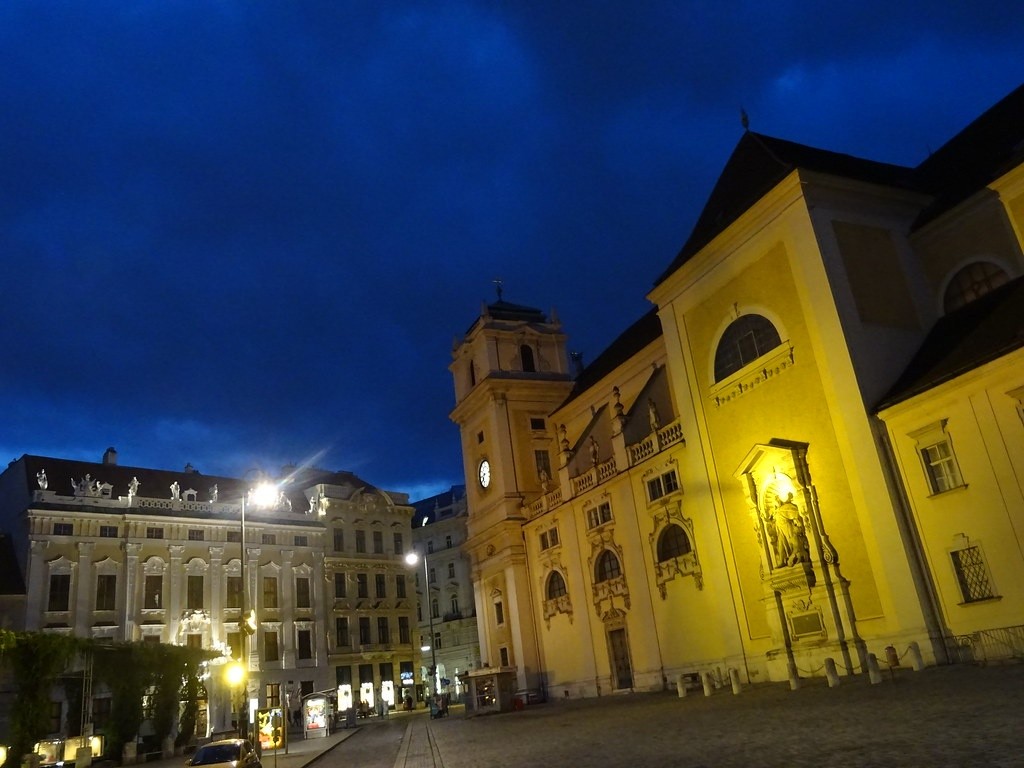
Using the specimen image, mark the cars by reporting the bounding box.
[186,738,263,768]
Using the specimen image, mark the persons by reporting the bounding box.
[770,497,807,567]
[358,698,369,718]
[406,694,413,712]
[287,707,302,729]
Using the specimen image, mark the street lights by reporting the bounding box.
[239,468,280,739]
[405,553,438,697]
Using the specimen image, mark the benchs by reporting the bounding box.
[180,745,200,755]
[138,750,165,763]
